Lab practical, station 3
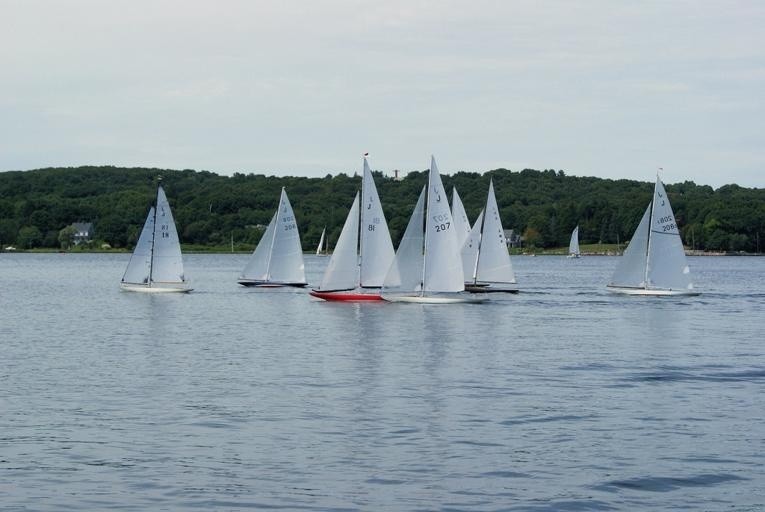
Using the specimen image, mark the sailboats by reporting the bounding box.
[120,175,195,294]
[565,225,582,258]
[606,167,704,302]
